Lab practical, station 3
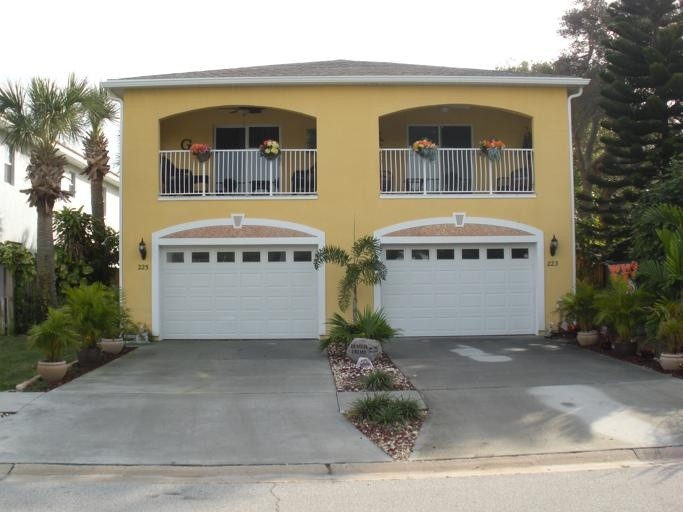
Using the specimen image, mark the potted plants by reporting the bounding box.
[553,277,601,348]
[591,274,654,358]
[643,299,682,372]
[62,277,145,368]
[27,308,77,386]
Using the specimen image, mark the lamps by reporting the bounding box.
[550,234,558,256]
[139,237,146,260]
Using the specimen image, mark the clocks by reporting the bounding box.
[181,139,191,150]
